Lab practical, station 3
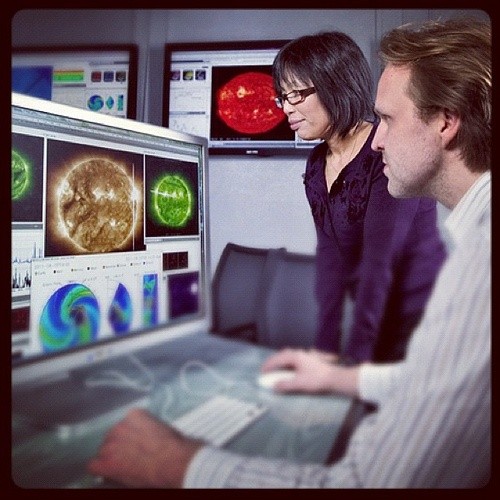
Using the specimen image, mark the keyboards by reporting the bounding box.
[175,393,268,449]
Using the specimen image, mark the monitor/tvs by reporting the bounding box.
[11,91,213,386]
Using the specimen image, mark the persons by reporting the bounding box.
[271,29,448,417]
[89,18,492,489]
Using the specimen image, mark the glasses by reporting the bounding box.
[274,87,317,109]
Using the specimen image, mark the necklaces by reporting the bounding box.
[325,126,359,179]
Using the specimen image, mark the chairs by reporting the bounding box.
[211,242,288,346]
[255,248,318,345]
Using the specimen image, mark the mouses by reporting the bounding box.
[258,368,295,388]
